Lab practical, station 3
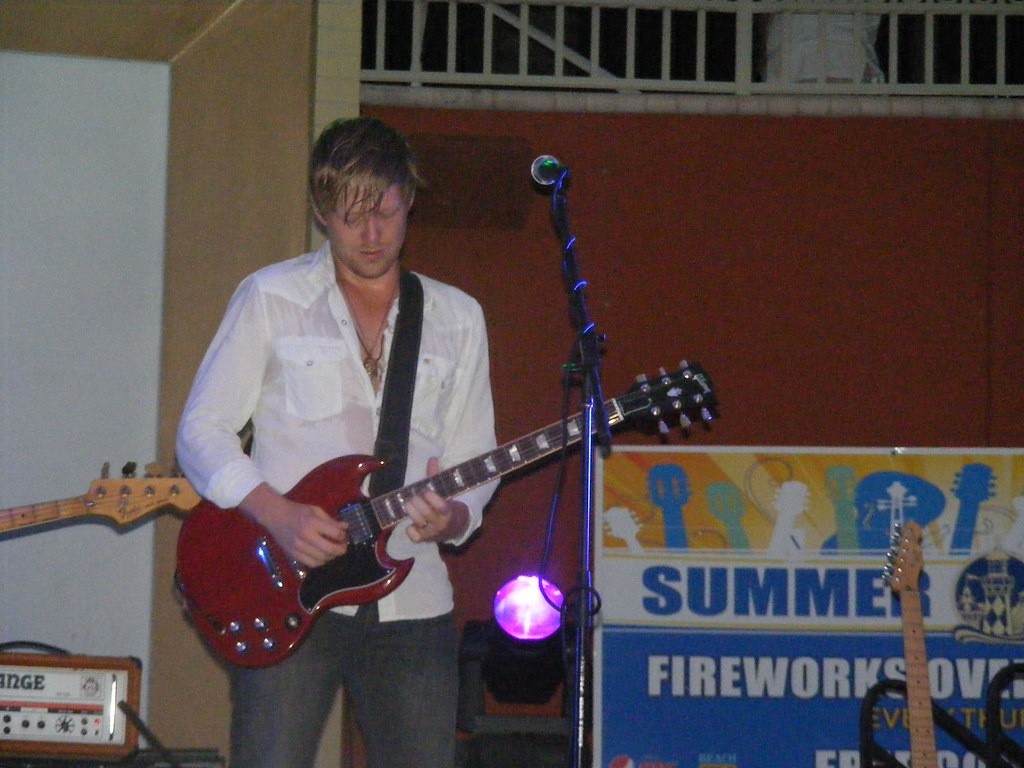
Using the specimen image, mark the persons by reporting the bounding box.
[174,116,499,768]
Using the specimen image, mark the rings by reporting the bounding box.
[423,520,428,529]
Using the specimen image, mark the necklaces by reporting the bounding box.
[340,269,399,379]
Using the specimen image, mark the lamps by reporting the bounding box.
[458,572,591,736]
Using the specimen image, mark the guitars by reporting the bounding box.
[173,357,722,673]
[2,460,201,540]
[884,518,941,767]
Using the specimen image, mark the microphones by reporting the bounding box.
[531,154,569,184]
